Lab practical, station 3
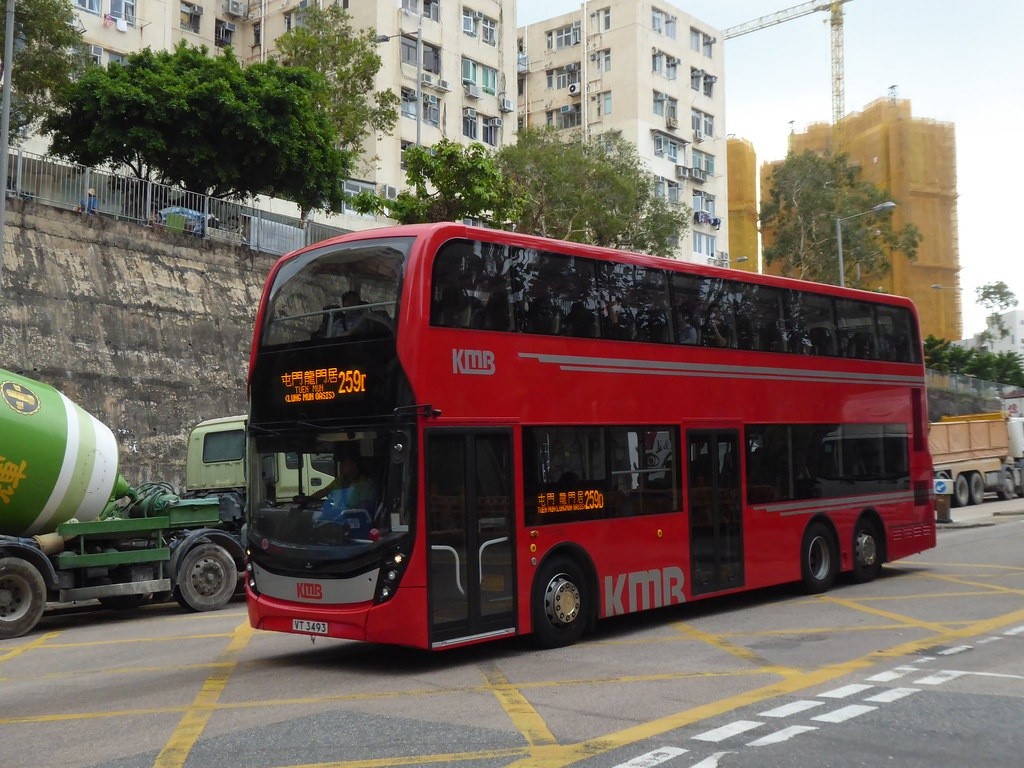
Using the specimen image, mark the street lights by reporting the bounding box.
[835,202,897,288]
[374,28,425,199]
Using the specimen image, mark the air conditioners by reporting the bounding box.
[466,85,482,99]
[409,73,452,99]
[86,45,103,58]
[491,118,503,128]
[708,36,717,43]
[676,167,729,269]
[561,103,577,114]
[653,48,718,84]
[382,184,400,202]
[658,93,669,101]
[427,148,438,156]
[464,108,476,119]
[501,98,514,113]
[299,0,316,9]
[667,116,705,143]
[565,64,577,73]
[472,11,483,20]
[191,0,244,32]
[567,82,580,97]
[665,14,676,22]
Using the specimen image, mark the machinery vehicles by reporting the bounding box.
[0,365,341,640]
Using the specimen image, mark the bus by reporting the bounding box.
[243,220,937,653]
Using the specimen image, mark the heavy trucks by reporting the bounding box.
[926,411,1024,508]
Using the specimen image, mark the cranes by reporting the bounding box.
[719,0,855,203]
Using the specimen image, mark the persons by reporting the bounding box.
[531,471,601,526]
[317,292,371,340]
[675,309,700,347]
[75,188,96,215]
[424,479,475,545]
[838,332,850,359]
[851,331,867,358]
[519,273,598,336]
[430,269,490,330]
[636,294,667,342]
[481,274,511,328]
[208,211,220,229]
[601,302,633,339]
[290,453,385,540]
[702,302,731,348]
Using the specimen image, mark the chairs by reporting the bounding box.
[447,288,911,363]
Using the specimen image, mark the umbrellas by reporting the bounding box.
[159,203,200,223]
